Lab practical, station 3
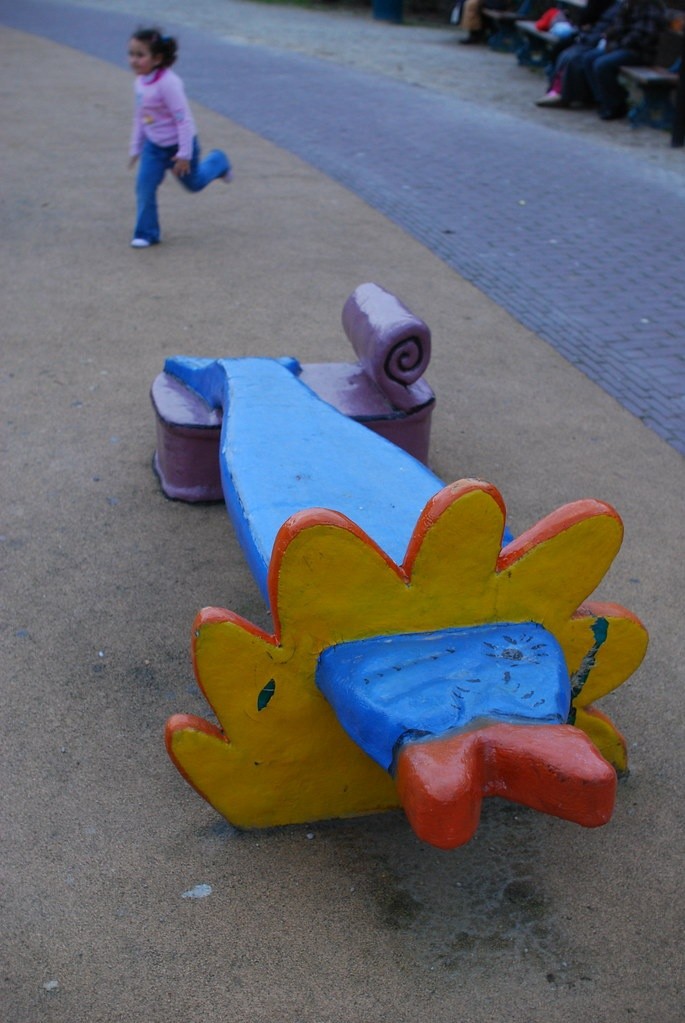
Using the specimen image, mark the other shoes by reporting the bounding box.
[131,238,149,248]
[601,110,626,120]
[223,171,233,183]
[535,94,562,106]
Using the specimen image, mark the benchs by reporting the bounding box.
[482,0,685,146]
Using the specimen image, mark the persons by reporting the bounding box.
[458,0,684,120]
[127,28,234,248]
